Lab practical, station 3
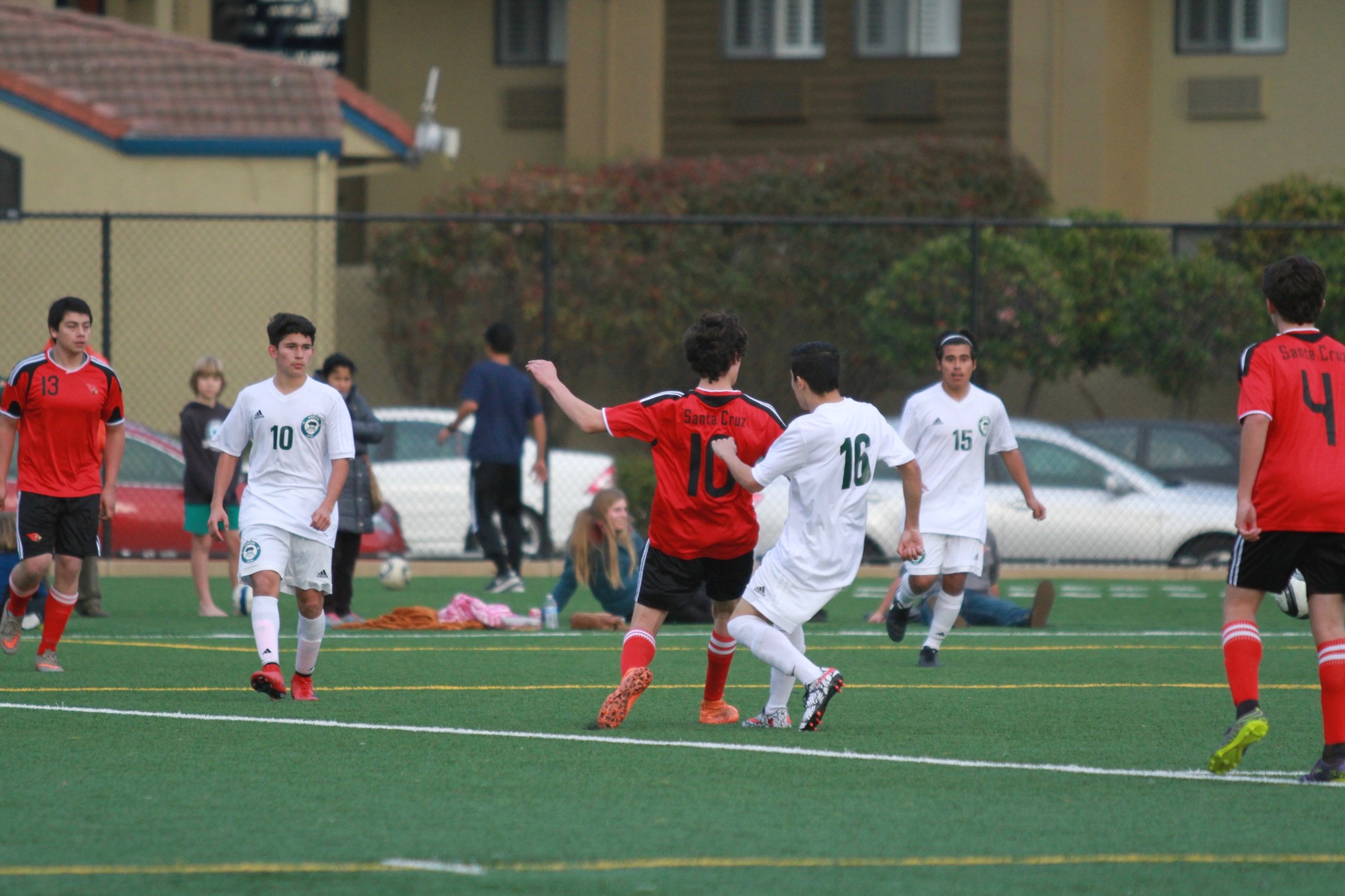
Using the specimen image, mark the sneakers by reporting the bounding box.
[36,650,65,673]
[1301,754,1345,786]
[699,697,741,723]
[597,666,653,729]
[343,614,366,625]
[886,587,915,643]
[918,646,942,668]
[798,667,846,731]
[324,613,344,629]
[0,598,24,655]
[251,662,287,700]
[742,707,792,729]
[486,571,525,594]
[1209,708,1269,776]
[291,671,319,702]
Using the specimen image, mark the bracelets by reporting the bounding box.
[447,423,457,434]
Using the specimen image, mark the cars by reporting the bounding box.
[1055,421,1239,485]
[746,417,1239,566]
[361,407,614,561]
[0,379,410,557]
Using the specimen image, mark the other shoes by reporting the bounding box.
[82,605,105,618]
[1029,580,1054,630]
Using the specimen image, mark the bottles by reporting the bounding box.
[544,594,559,630]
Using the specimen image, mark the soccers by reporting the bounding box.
[1274,567,1310,620]
[378,556,413,592]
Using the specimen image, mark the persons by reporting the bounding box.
[207,313,355,701]
[525,313,787,728]
[435,321,547,594]
[177,358,240,617]
[541,488,715,626]
[868,529,1054,630]
[0,297,126,672]
[312,352,385,628]
[886,330,1047,667]
[710,341,926,732]
[1208,254,1345,780]
